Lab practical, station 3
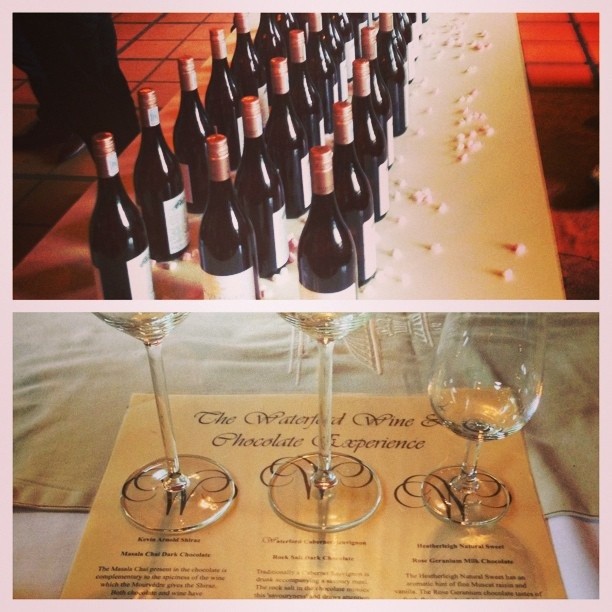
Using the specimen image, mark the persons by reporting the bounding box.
[15,14,140,165]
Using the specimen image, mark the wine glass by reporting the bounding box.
[421,312,545,527]
[92,312,234,535]
[269,312,383,534]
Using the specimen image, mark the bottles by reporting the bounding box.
[393,13,407,59]
[134,89,191,260]
[353,58,390,223]
[322,13,348,102]
[199,135,261,301]
[266,57,314,218]
[233,97,289,278]
[377,13,406,137]
[205,28,244,170]
[361,26,397,170]
[173,58,215,213]
[90,131,153,301]
[307,13,340,117]
[402,14,412,44]
[331,101,379,285]
[411,14,420,41]
[338,13,356,83]
[231,13,270,127]
[281,13,299,37]
[288,29,326,146]
[299,144,359,299]
[253,13,285,63]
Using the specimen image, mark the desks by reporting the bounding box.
[12,12,566,301]
